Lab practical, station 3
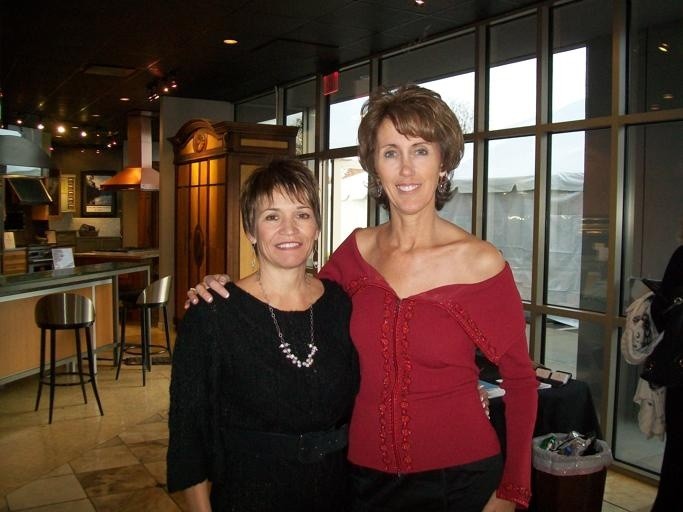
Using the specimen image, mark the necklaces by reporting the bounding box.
[258,272,318,368]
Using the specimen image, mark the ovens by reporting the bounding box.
[27,258,54,273]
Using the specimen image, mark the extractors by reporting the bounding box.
[0,127,64,181]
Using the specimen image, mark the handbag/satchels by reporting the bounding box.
[640,334,683,387]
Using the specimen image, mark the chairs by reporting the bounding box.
[33,273,174,425]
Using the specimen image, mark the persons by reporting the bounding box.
[650,246,682,511]
[184,81,539,512]
[167,155,360,512]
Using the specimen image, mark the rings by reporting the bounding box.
[189,287,197,295]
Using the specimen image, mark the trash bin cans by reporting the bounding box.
[531,432,614,512]
